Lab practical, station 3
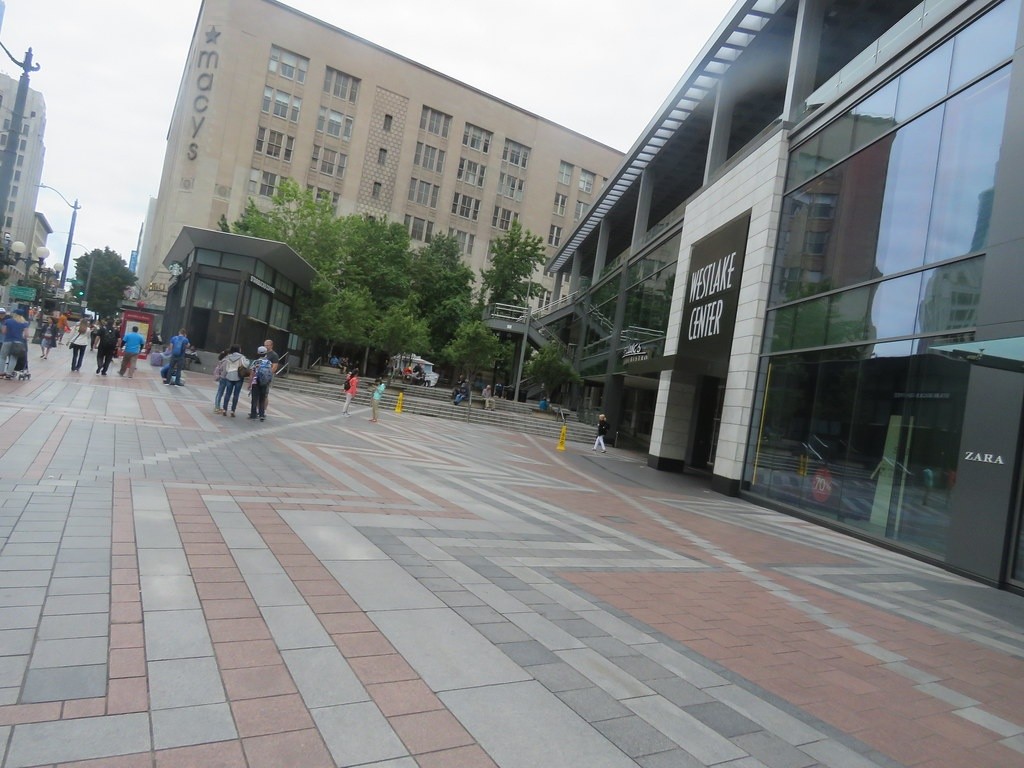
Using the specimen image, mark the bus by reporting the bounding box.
[41,298,81,324]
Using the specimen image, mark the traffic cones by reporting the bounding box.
[556,426,566,451]
[394,392,404,413]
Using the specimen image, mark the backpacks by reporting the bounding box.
[213,360,225,381]
[258,365,272,387]
[344,377,353,390]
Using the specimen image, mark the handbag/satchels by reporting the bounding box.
[238,359,250,377]
[9,342,23,355]
[68,342,74,348]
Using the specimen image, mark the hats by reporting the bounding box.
[13,309,24,315]
[0,308,6,314]
[257,346,267,355]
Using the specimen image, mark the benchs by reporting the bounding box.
[462,398,486,409]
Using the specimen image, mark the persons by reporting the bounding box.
[330,355,352,374]
[593,414,608,452]
[341,368,359,416]
[0,305,121,380]
[246,345,272,420]
[250,340,278,416]
[944,469,957,506]
[482,385,495,411]
[412,363,425,385]
[146,328,190,386]
[923,467,933,506]
[214,344,249,417]
[495,382,511,400]
[117,326,144,378]
[451,375,468,405]
[539,397,548,410]
[369,379,385,422]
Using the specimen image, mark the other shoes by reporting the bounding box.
[96,366,102,374]
[230,411,235,417]
[223,409,227,416]
[100,372,107,375]
[260,417,264,421]
[343,412,351,417]
[369,417,377,422]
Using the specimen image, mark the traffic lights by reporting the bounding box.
[78,290,84,298]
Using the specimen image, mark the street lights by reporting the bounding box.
[31,261,66,344]
[34,182,81,310]
[11,241,50,286]
[73,242,95,316]
